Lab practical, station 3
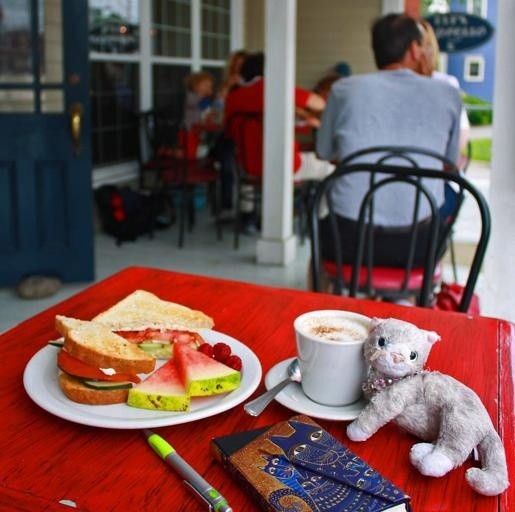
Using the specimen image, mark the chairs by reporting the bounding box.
[307,144,491,314]
[132,106,262,249]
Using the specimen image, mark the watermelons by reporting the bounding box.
[127,341,242,413]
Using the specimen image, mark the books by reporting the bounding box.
[208,413,412,512]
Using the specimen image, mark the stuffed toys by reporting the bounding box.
[347,316,509,496]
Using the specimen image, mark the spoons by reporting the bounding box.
[243,356,302,418]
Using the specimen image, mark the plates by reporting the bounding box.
[264,355,369,421]
[23,327,263,430]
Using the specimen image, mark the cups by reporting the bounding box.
[293,310,374,407]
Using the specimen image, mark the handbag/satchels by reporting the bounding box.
[95,184,175,243]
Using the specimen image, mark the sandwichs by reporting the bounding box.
[89,289,214,360]
[47,315,157,406]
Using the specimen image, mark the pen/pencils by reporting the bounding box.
[144,428,232,512]
